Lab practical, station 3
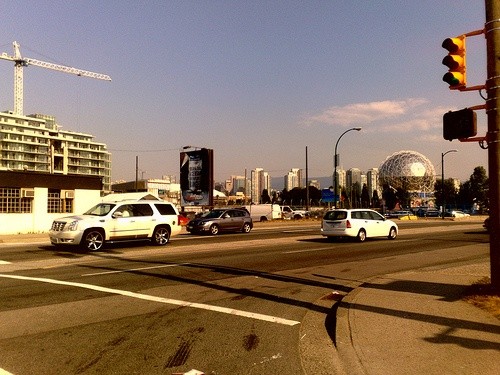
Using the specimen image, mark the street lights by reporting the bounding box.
[441,149,457,220]
[334,128,361,208]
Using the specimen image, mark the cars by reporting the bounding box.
[50,193,181,253]
[320,208,399,242]
[387,206,448,219]
[178,207,254,236]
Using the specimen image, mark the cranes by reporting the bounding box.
[0,41,110,118]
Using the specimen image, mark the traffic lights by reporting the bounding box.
[442,34,467,90]
[443,109,477,140]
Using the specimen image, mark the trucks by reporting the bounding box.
[245,204,308,222]
